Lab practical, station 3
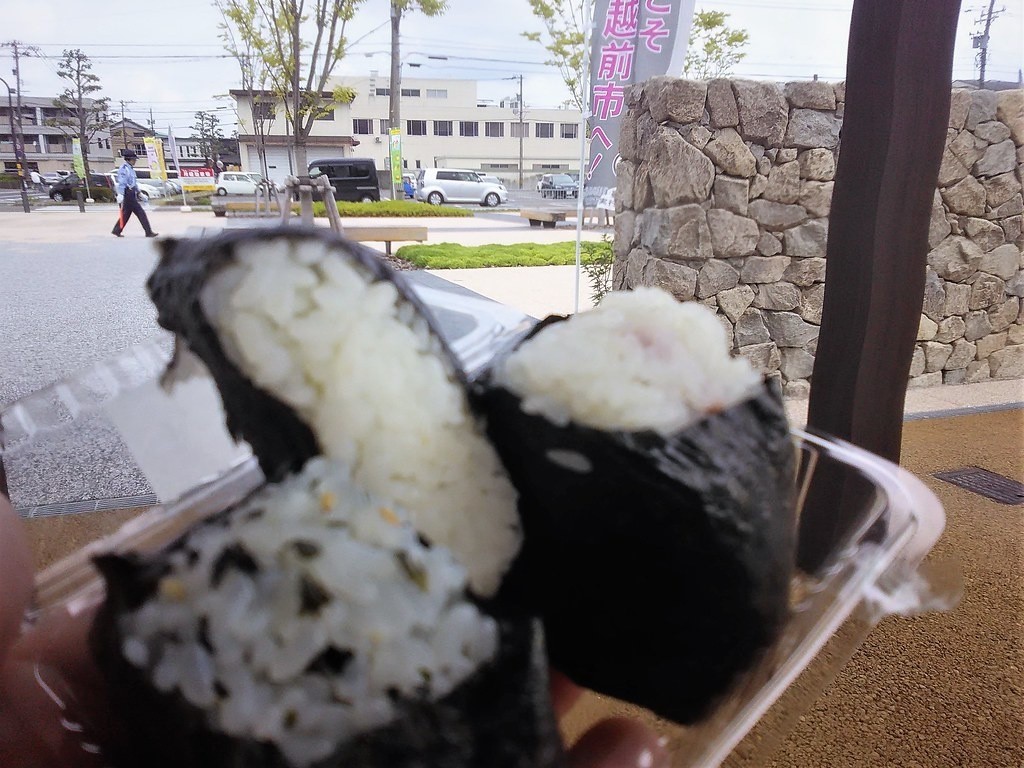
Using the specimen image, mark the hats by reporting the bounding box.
[124,150,139,160]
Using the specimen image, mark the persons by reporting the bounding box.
[30,169,47,193]
[0,494,665,768]
[112,150,158,237]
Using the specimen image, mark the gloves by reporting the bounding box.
[117,194,124,203]
[138,192,148,202]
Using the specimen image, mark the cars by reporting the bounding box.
[5,168,185,203]
[403,172,418,190]
[480,176,502,186]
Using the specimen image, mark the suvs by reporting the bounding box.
[536,174,579,199]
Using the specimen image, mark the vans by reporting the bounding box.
[415,167,508,207]
[217,171,281,196]
[279,157,381,204]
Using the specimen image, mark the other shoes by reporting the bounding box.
[111,231,124,237]
[146,232,158,237]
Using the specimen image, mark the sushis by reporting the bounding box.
[83,226,799,768]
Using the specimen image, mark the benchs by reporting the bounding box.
[221,228,425,254]
[520,209,615,228]
